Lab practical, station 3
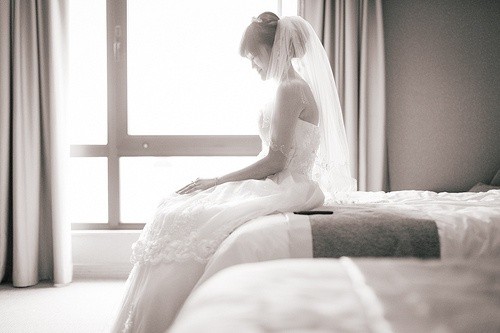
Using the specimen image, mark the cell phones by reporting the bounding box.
[293,211,333,215]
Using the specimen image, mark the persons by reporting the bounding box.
[114,10,353,333]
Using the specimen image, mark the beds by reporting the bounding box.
[167,258,500,333]
[193,190,499,289]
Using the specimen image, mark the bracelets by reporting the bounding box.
[214,176,218,186]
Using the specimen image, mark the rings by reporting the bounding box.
[194,182,197,185]
